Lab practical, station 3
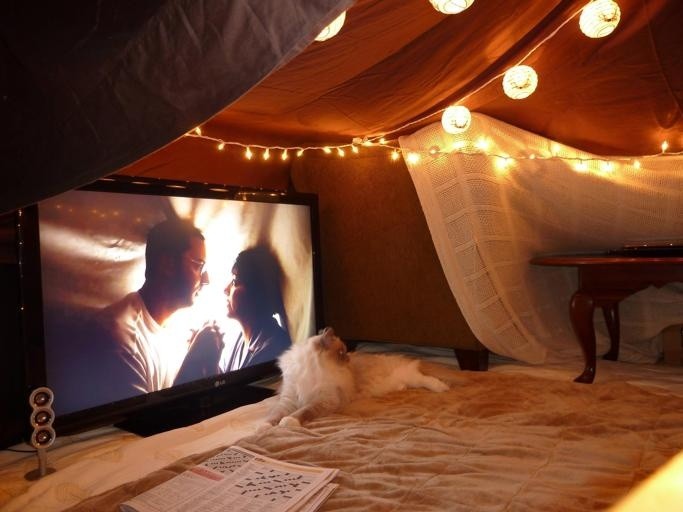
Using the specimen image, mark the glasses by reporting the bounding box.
[186,256,206,276]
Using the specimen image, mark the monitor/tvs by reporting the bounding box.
[15,174,325,445]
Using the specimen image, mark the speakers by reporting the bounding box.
[21,381,57,481]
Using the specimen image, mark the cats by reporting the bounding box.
[254,326,453,435]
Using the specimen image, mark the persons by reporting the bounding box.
[49,218,217,414]
[187,245,290,381]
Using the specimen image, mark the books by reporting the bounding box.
[119,444,341,512]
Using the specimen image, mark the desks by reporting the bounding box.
[527,245,683,384]
